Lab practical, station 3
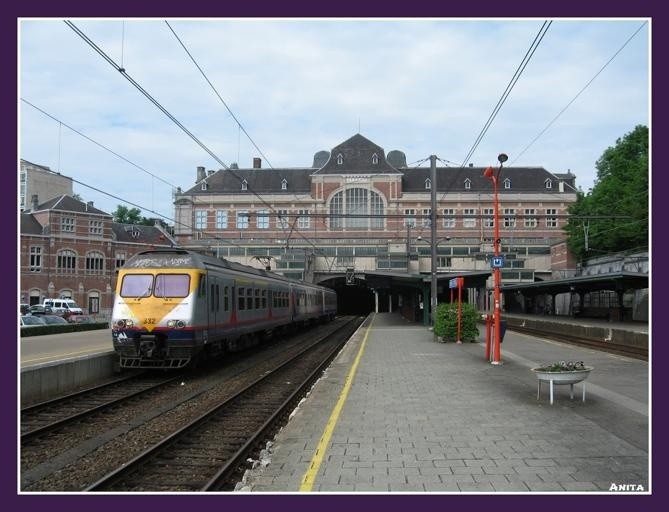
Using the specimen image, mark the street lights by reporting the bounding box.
[417,235,452,328]
[483,153,508,366]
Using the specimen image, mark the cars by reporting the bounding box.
[20,303,89,326]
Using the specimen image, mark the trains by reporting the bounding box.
[111,248,337,374]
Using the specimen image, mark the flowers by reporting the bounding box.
[537,359,588,371]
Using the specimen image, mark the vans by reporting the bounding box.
[43,298,83,315]
